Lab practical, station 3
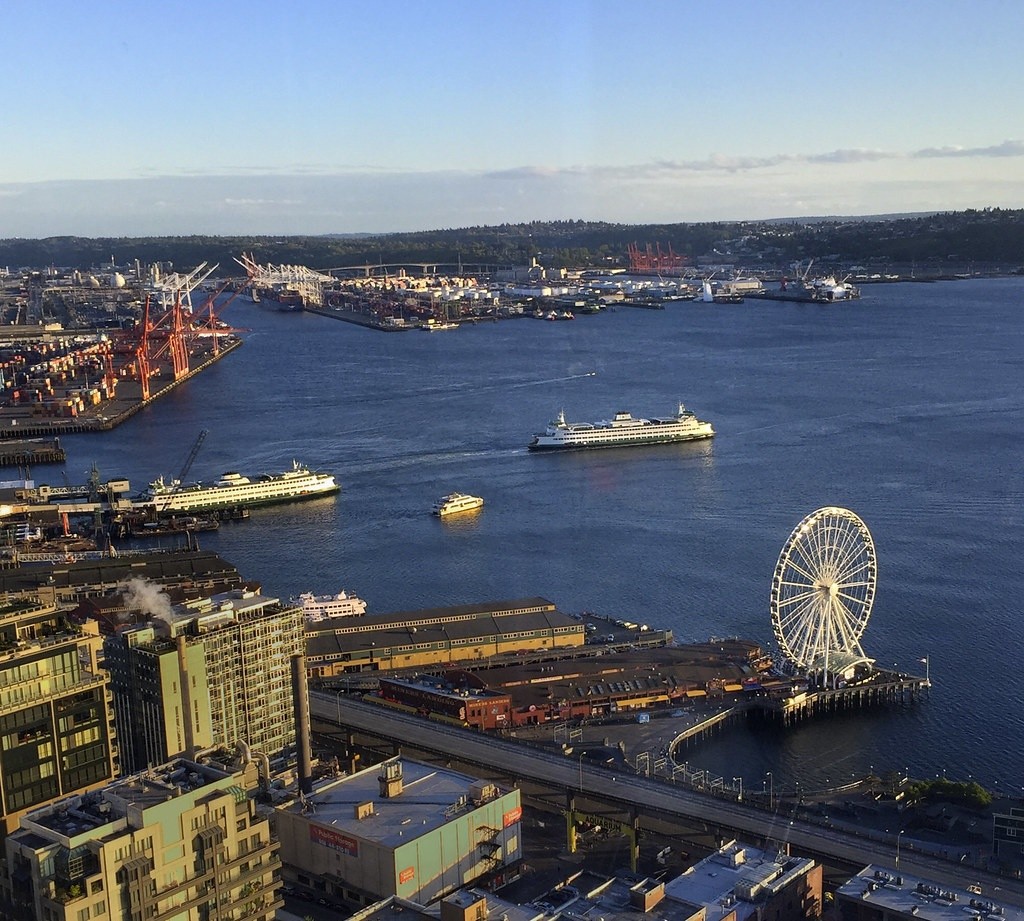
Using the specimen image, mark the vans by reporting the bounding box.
[635,711,650,723]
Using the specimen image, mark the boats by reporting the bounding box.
[135,458,342,517]
[285,589,367,624]
[429,492,485,517]
[526,399,718,452]
[420,324,459,331]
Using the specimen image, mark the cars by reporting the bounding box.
[574,613,615,644]
[670,709,689,719]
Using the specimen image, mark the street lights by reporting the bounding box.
[897,831,905,868]
[476,699,485,733]
[336,690,344,726]
[766,772,772,810]
[580,752,586,793]
